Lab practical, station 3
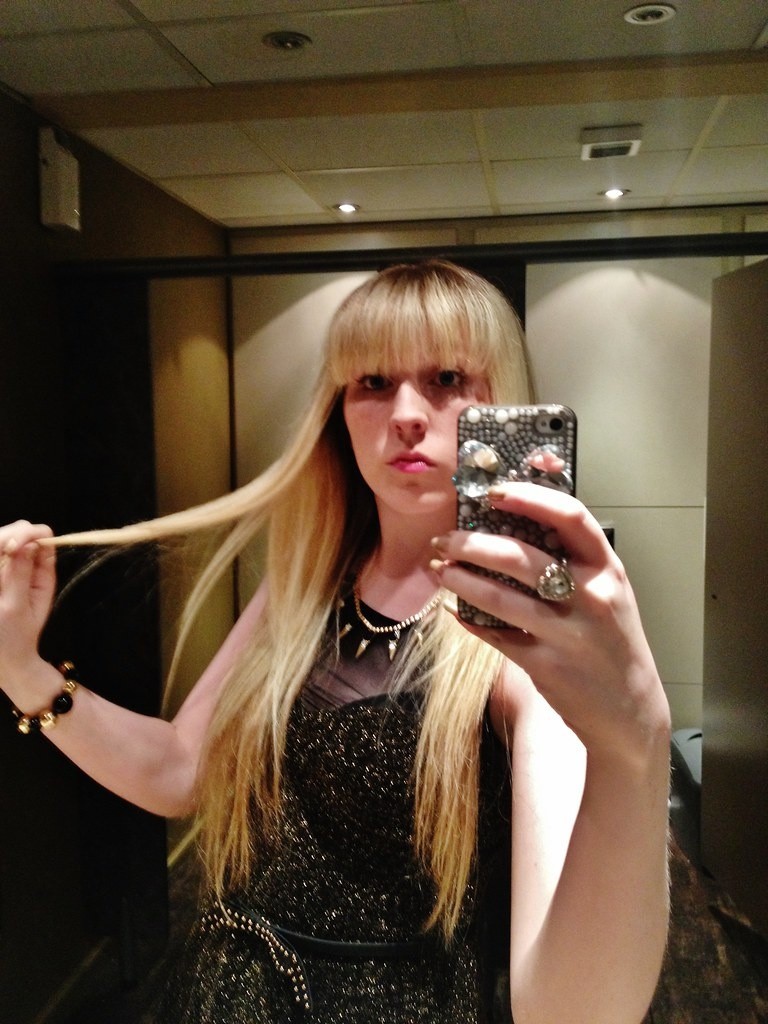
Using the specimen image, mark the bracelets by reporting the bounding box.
[8,659,77,736]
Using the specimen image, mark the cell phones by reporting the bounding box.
[456,404,579,626]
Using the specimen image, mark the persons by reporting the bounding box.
[1,262,671,1024]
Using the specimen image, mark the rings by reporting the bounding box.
[539,555,575,605]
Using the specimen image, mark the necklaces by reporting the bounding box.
[353,547,449,634]
